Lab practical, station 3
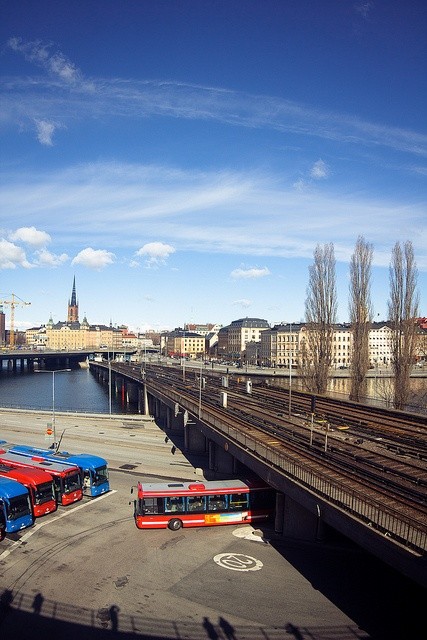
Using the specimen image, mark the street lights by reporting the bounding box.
[56,425,78,452]
[34,368,72,454]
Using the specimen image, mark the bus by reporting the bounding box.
[0,449,83,505]
[0,466,57,516]
[0,478,33,532]
[0,439,109,497]
[128,479,276,530]
